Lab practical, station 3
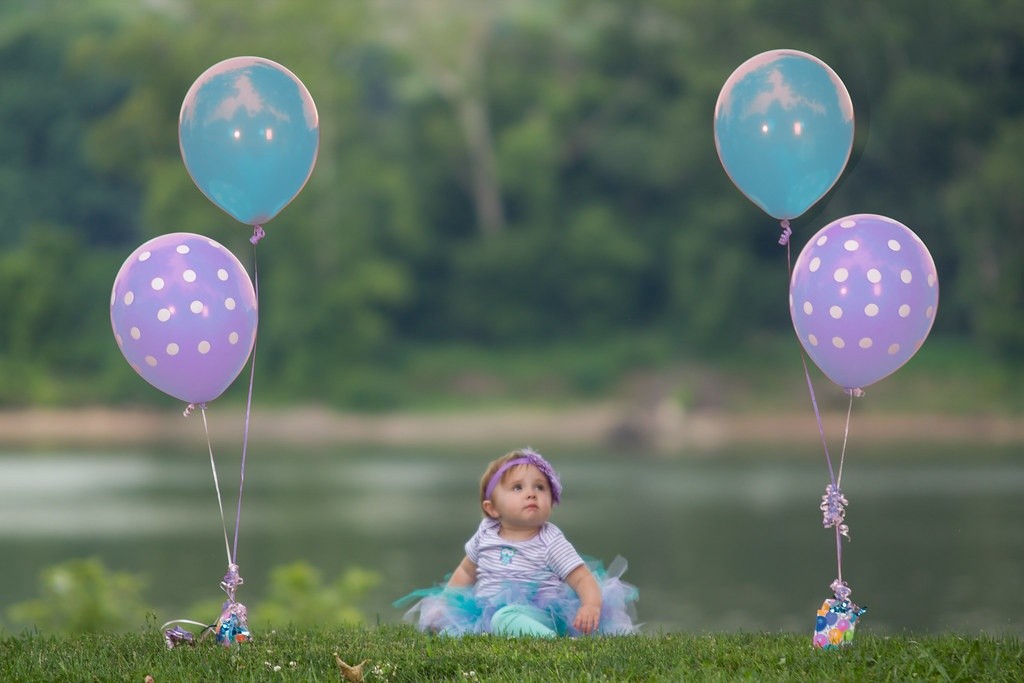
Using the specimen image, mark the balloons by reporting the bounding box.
[108,230,258,404]
[712,50,856,227]
[786,211,940,397]
[177,55,320,235]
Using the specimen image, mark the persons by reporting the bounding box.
[395,446,644,644]
